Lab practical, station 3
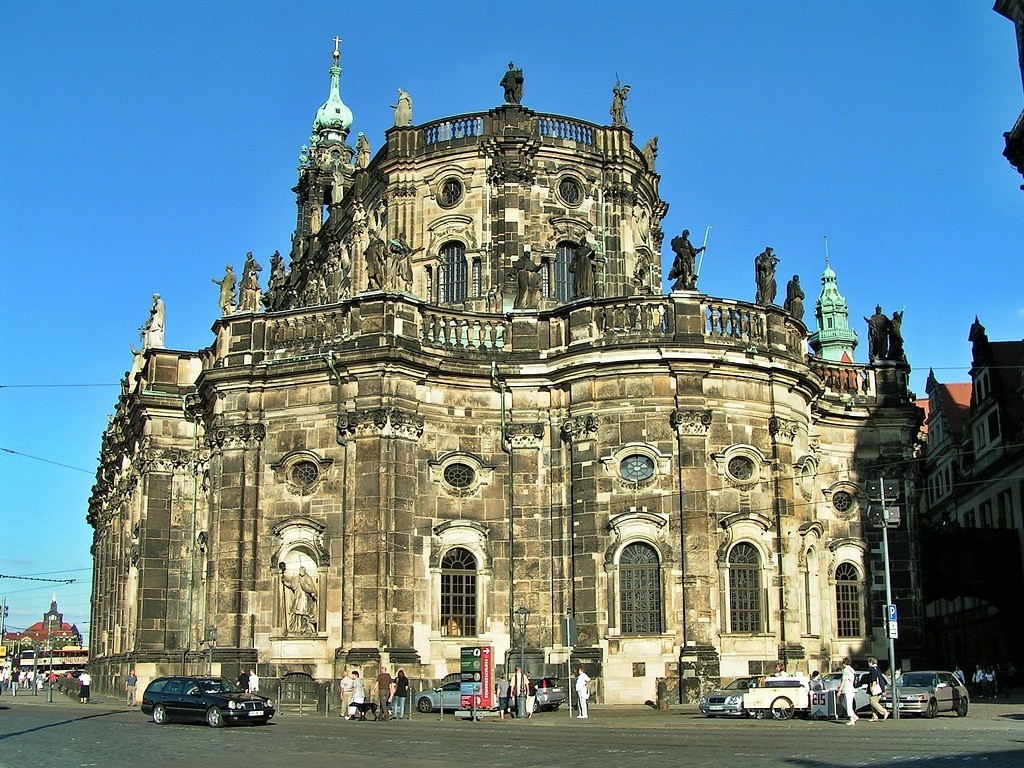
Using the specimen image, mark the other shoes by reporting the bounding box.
[133,704,137,707]
[853,716,859,722]
[846,722,855,725]
[127,704,130,706]
[883,712,890,721]
[577,716,588,718]
[867,717,878,722]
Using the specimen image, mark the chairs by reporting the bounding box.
[171,682,179,691]
[455,685,459,691]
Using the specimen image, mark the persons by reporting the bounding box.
[509,251,541,310]
[78,671,91,703]
[862,304,904,361]
[775,663,784,671]
[500,61,524,104]
[125,670,138,706]
[363,229,425,293]
[211,250,285,315]
[576,667,591,718]
[972,661,1016,699]
[613,81,631,124]
[569,236,595,300]
[954,666,965,685]
[668,229,706,283]
[497,666,537,718]
[281,566,318,633]
[866,657,890,722]
[237,667,259,694]
[755,247,806,319]
[644,136,659,172]
[120,293,166,395]
[340,667,409,720]
[836,658,859,725]
[390,88,412,126]
[0,666,73,696]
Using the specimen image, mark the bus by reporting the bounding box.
[20,648,89,683]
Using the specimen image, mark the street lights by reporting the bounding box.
[46,631,57,703]
[864,477,901,719]
[0,595,9,646]
[514,605,531,717]
[207,636,216,677]
[30,640,42,697]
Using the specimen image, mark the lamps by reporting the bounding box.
[846,400,856,411]
[742,346,758,355]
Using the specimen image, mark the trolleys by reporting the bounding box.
[742,676,840,722]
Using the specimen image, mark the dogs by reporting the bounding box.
[349,701,384,722]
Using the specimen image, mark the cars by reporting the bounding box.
[507,676,565,714]
[698,674,776,720]
[878,670,970,719]
[818,671,892,720]
[414,681,500,713]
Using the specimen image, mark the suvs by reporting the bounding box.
[141,674,276,728]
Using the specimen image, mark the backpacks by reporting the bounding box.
[845,668,860,689]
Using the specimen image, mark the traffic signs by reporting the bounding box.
[460,645,494,710]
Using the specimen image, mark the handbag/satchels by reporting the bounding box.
[870,684,882,696]
